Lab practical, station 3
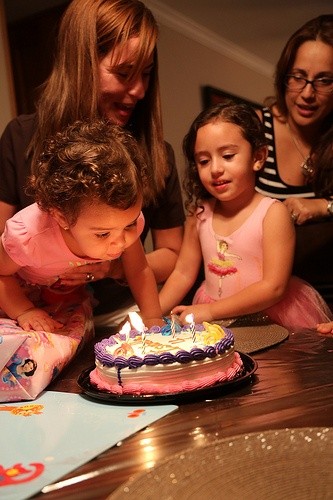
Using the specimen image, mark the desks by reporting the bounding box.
[0,314,333,500]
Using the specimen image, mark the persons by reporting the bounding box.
[316,321,333,335]
[157,96,333,330]
[0,119,167,335]
[253,15,333,316]
[0,0,187,334]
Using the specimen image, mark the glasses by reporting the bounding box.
[284,77,333,96]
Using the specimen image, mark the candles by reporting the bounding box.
[126,311,196,354]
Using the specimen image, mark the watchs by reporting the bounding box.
[327,201,333,215]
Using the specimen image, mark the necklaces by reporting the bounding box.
[286,122,315,176]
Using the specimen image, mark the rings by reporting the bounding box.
[291,213,298,218]
[86,273,95,281]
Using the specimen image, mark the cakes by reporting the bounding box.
[89,322,243,395]
